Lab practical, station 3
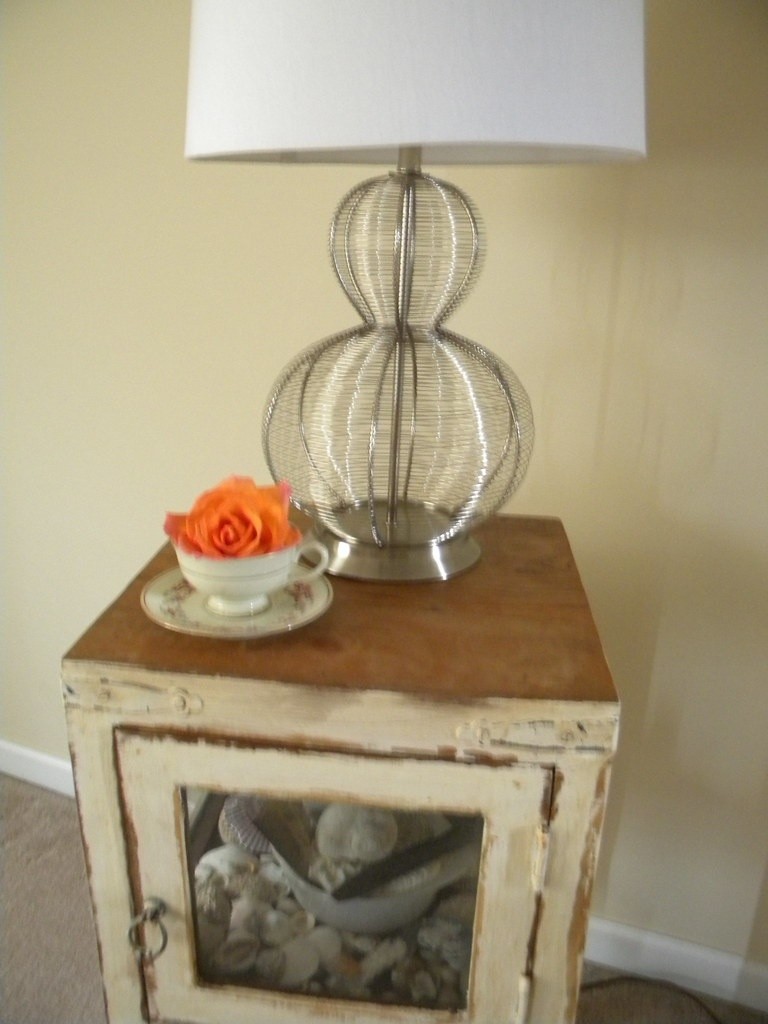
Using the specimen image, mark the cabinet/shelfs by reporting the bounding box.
[60,515,621,1024]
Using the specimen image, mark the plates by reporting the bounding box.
[140,557,335,642]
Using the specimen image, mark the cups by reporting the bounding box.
[169,513,329,618]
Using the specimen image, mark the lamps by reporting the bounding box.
[183,1,650,584]
